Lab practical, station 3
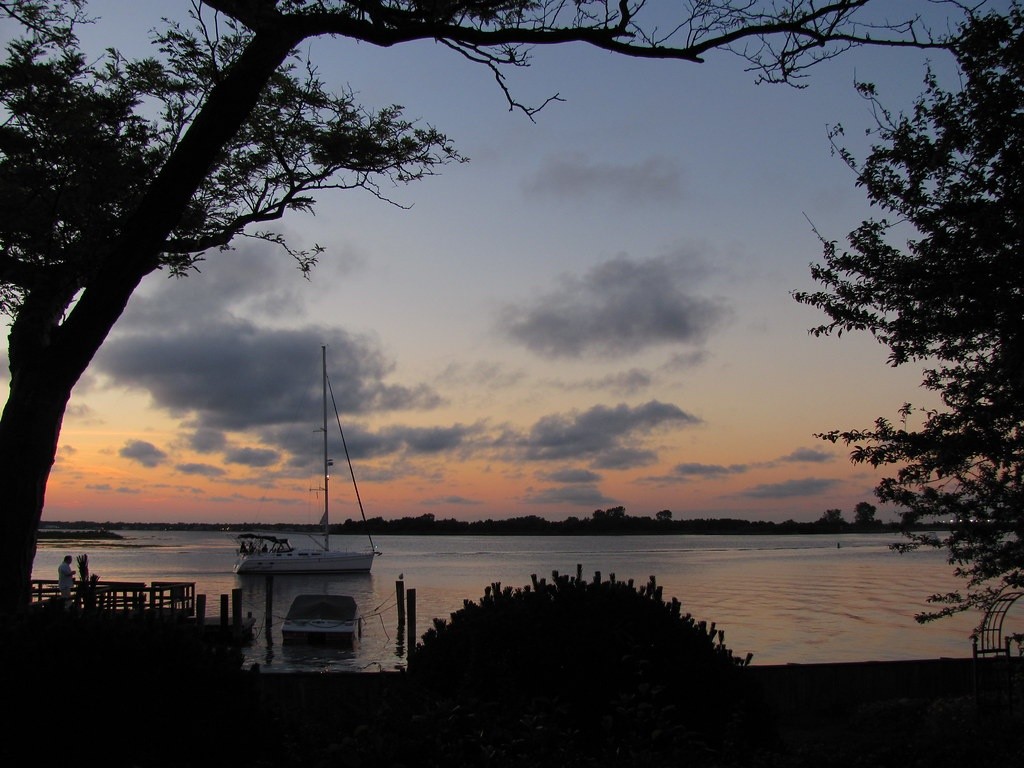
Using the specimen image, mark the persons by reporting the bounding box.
[249,542,256,552]
[58,555,76,597]
[240,541,249,555]
[262,544,268,552]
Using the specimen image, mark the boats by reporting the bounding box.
[281,594,358,650]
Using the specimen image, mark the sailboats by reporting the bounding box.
[231,345,381,579]
